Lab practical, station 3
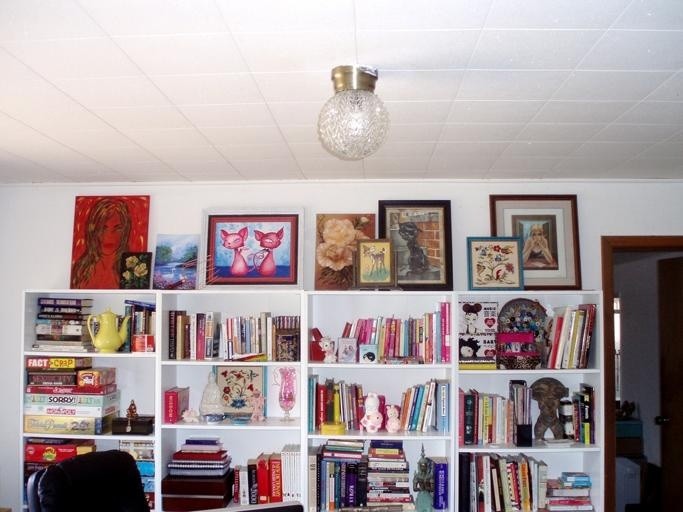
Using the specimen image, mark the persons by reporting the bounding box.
[70,196,132,289]
[199,371,227,416]
[522,223,555,266]
[413,459,435,512]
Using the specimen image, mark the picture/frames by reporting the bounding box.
[352,193,582,291]
[195,206,305,291]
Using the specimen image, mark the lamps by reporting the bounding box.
[315,63,388,164]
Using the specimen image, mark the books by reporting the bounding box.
[310,375,449,434]
[459,379,595,447]
[160,436,301,512]
[308,440,414,512]
[165,307,301,364]
[547,302,596,368]
[459,453,594,512]
[459,301,499,369]
[312,302,450,363]
[163,382,191,423]
[427,456,448,510]
[22,296,155,510]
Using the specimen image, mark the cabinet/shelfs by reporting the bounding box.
[14,290,605,511]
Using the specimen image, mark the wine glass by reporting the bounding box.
[278,368,296,422]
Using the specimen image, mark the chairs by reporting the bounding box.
[26,445,304,512]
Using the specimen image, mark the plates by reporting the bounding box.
[544,439,576,448]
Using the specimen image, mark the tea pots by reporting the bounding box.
[86,309,131,353]
